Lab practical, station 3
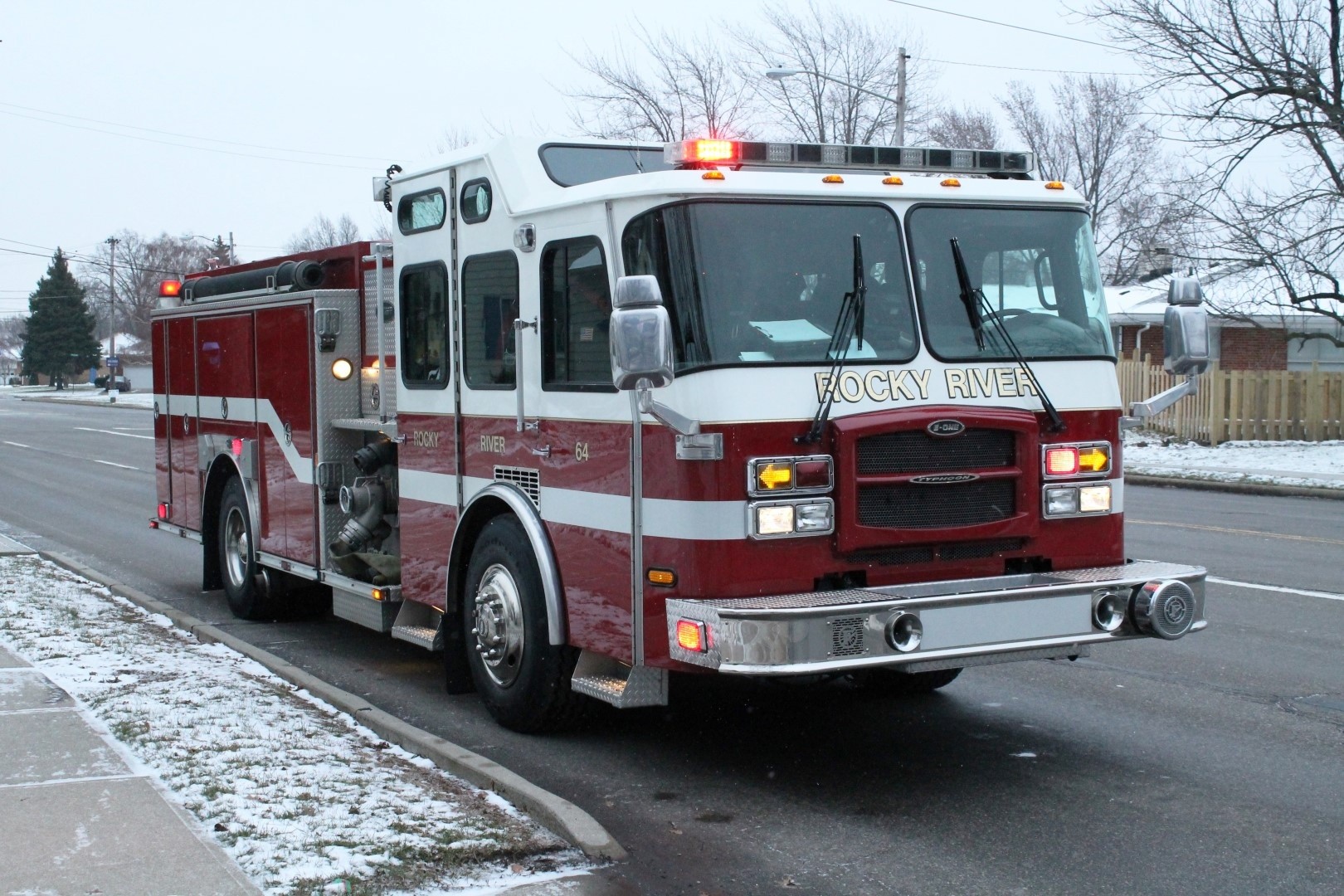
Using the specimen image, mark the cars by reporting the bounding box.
[94,375,109,387]
[105,376,131,391]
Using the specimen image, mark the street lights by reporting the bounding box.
[180,232,234,266]
[60,255,115,405]
[766,48,905,146]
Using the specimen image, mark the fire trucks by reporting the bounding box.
[148,139,1210,734]
